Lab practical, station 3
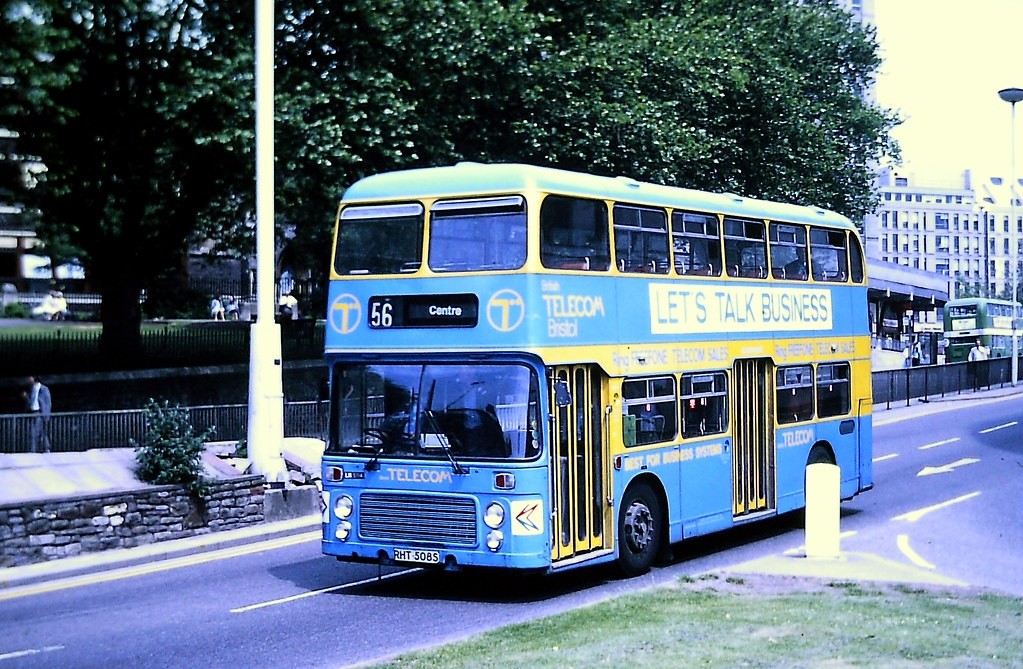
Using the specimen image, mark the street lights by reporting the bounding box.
[997,88,1023,387]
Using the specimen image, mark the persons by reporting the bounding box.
[210,292,226,320]
[226,294,239,320]
[22,373,51,453]
[967,337,990,361]
[47,286,66,320]
[876,332,924,367]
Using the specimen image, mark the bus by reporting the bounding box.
[943,297,1023,363]
[318,162,875,581]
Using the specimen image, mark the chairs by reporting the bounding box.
[536,229,847,283]
[382,404,726,456]
